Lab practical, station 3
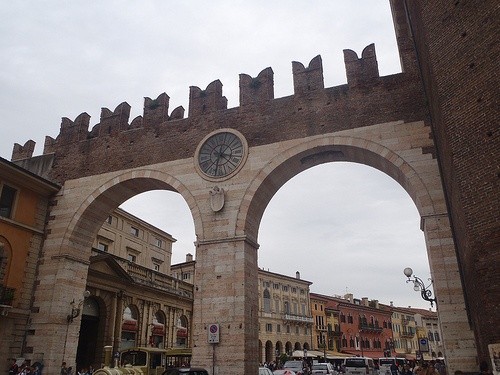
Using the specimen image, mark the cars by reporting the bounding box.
[161,366,209,375]
[259,367,295,375]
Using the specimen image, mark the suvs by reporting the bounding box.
[282,361,334,375]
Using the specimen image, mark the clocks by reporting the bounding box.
[193,127,249,183]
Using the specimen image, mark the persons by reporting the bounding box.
[390,358,445,375]
[8,359,38,375]
[61,362,96,375]
[259,360,279,371]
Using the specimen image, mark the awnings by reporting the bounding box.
[286,351,401,359]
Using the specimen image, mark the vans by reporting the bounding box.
[343,357,375,375]
[379,357,407,375]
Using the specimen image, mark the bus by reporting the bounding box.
[92,346,192,375]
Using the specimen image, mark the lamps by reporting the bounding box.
[67,289,91,324]
[403,266,437,311]
